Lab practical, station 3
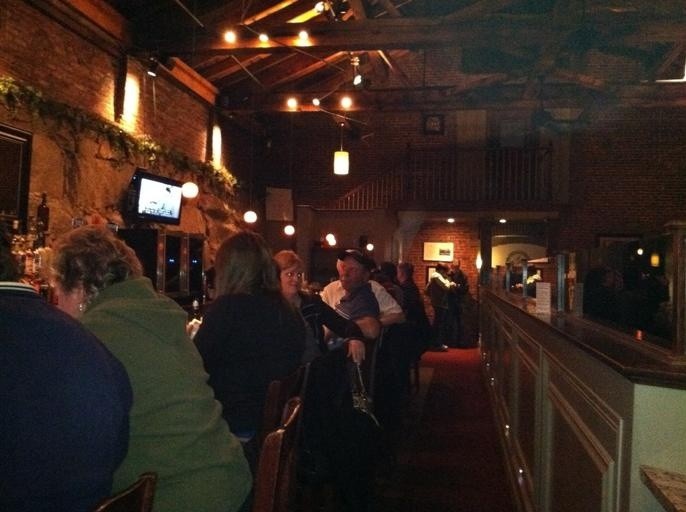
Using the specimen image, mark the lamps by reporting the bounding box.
[351,51,371,90]
[145,60,159,77]
[333,121,350,176]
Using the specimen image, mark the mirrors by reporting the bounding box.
[0,121,33,236]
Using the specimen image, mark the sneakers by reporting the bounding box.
[432,344,448,352]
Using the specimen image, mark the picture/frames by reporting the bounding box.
[594,232,645,265]
[426,266,437,285]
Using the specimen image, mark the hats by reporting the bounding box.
[337,250,373,271]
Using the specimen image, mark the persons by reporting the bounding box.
[0,227,133,512]
[273,248,369,369]
[514,265,537,296]
[316,246,470,423]
[51,228,255,510]
[190,228,306,475]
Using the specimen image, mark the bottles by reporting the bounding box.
[9,214,52,277]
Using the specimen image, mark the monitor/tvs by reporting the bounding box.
[123,167,185,225]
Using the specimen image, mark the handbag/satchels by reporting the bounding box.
[353,393,381,427]
